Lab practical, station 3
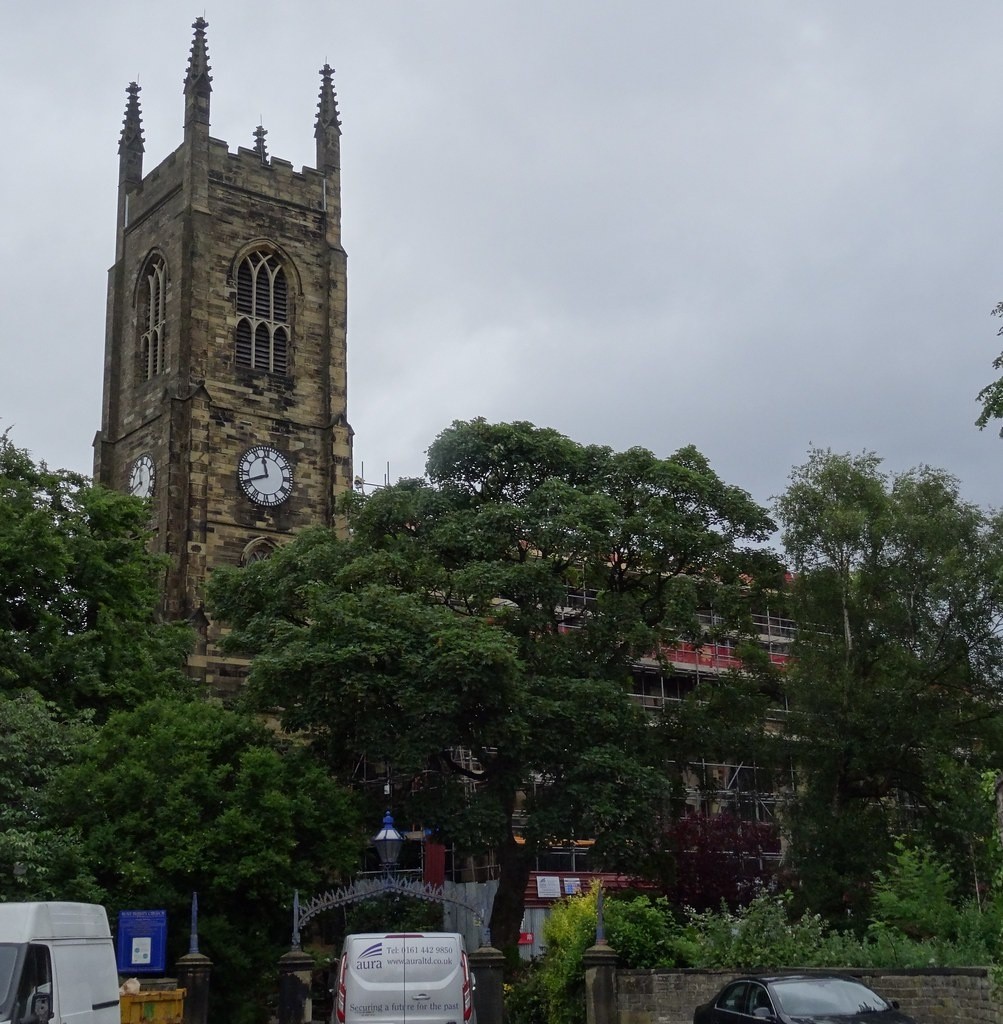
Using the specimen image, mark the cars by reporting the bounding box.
[692,971,913,1023]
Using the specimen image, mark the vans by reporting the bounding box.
[0,900,121,1024]
[331,934,479,1023]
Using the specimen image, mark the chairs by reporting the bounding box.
[735,994,743,1013]
[753,990,775,1017]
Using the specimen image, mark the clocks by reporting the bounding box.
[239,445,296,508]
[126,453,157,507]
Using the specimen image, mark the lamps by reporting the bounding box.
[372,810,403,864]
